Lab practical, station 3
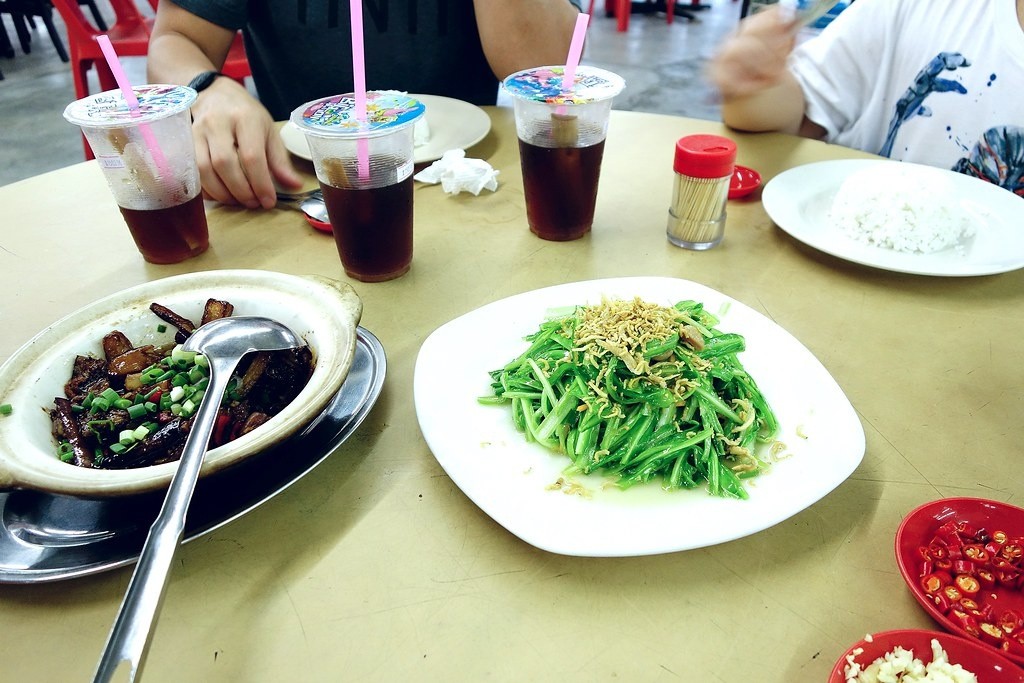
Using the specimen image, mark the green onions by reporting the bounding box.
[0,324,244,463]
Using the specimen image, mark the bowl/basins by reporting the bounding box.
[0,269,363,493]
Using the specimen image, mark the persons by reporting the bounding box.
[146,0,586,208]
[707,0,1024,198]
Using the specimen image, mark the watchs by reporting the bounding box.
[187,71,229,93]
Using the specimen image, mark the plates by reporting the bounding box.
[280,93,491,164]
[894,497,1024,664]
[828,629,1024,683]
[761,159,1024,277]
[411,275,865,558]
[305,212,333,232]
[727,164,762,200]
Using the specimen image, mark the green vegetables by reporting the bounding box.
[474,297,778,500]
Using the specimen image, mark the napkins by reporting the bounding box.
[413,150,497,197]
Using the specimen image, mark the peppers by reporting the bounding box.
[915,519,1024,660]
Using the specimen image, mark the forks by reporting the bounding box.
[276,188,331,223]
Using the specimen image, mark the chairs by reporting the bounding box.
[586,0,673,32]
[0,0,250,161]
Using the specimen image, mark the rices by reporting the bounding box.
[362,89,430,147]
[826,166,978,255]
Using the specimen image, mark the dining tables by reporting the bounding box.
[0,109,1024,683]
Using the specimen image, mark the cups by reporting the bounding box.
[291,90,426,282]
[64,84,209,264]
[666,134,738,250]
[502,66,626,242]
